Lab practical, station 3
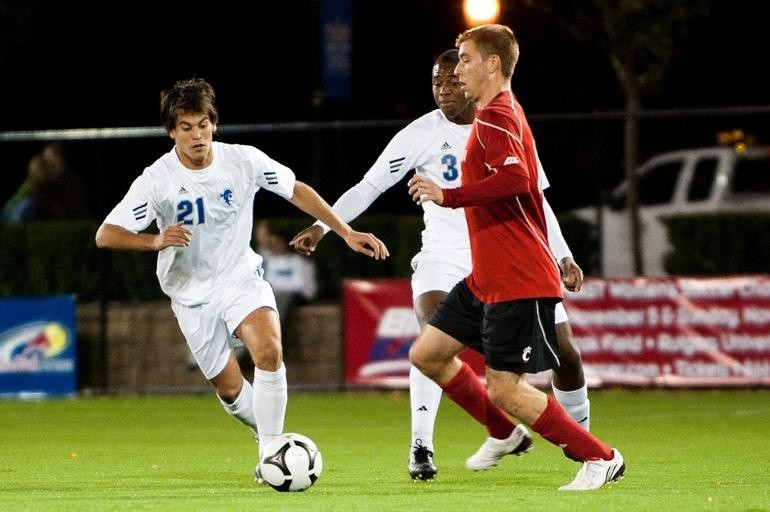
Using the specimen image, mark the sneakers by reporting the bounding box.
[254,463,269,485]
[557,449,627,492]
[408,437,437,479]
[465,423,535,469]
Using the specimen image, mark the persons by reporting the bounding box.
[1,143,88,223]
[96,78,391,486]
[177,217,317,375]
[286,47,591,483]
[407,25,627,489]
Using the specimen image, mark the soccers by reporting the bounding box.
[261,433,323,492]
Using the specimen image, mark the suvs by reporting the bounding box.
[564,146,770,275]
